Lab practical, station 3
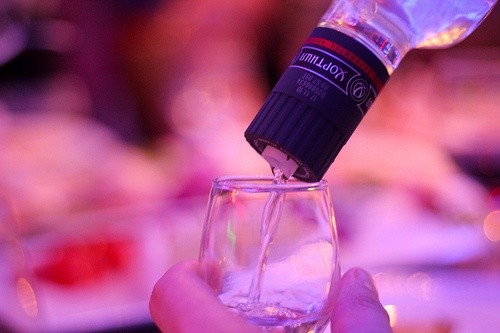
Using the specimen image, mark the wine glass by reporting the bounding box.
[198,176,341,333]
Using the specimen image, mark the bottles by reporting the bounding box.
[243,0,497,182]
